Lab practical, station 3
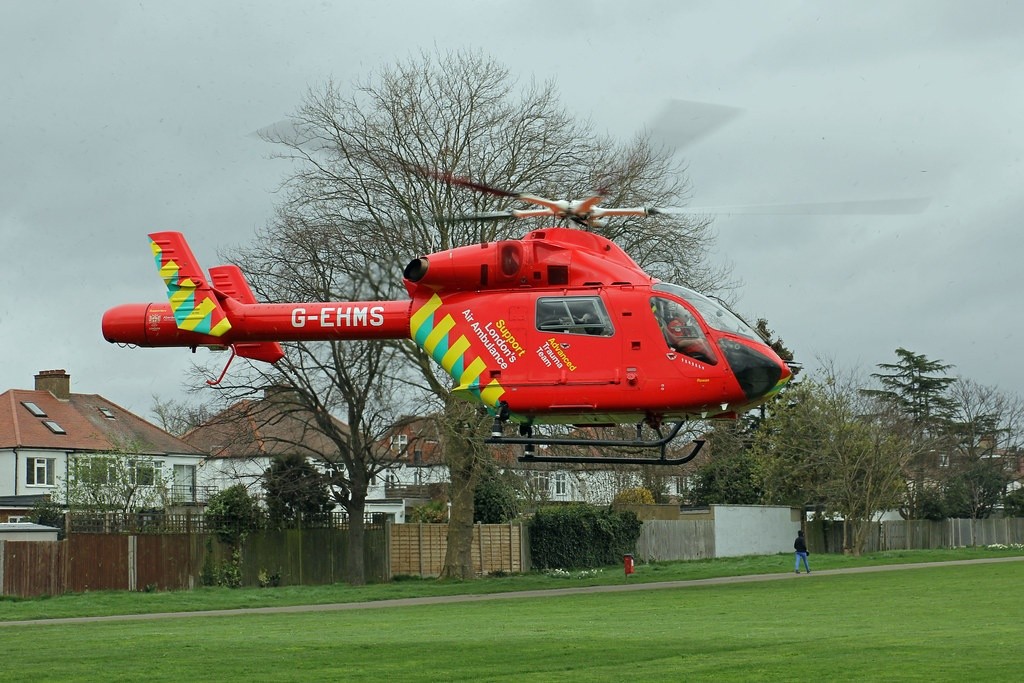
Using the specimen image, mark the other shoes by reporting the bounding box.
[796,572,800,574]
[807,569,811,573]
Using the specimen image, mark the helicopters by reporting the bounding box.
[103,99,935,466]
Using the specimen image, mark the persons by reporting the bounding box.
[794,531,811,574]
[668,307,717,364]
